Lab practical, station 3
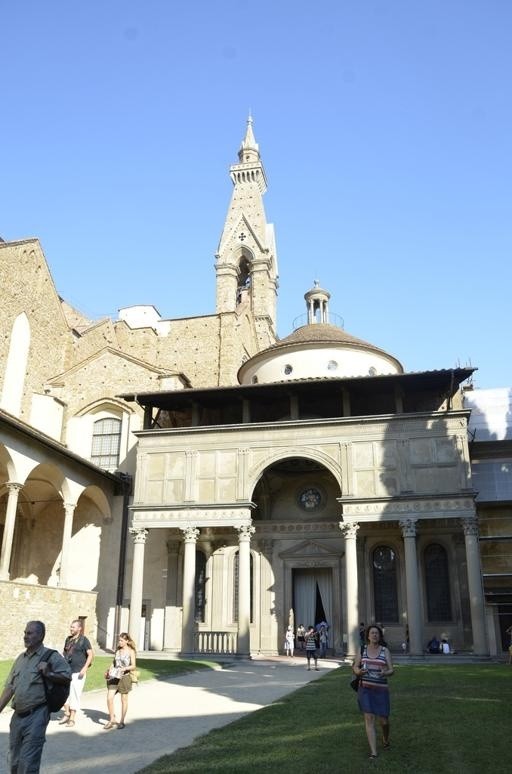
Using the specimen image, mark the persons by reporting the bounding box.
[427,636,438,651]
[296,623,307,649]
[439,641,449,655]
[57,619,94,725]
[1,617,74,774]
[360,622,367,645]
[283,624,295,656]
[506,625,512,666]
[405,625,409,644]
[317,626,329,658]
[304,625,321,672]
[377,623,387,645]
[350,627,396,762]
[101,631,137,730]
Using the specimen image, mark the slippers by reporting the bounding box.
[117,723,126,730]
[381,735,392,752]
[58,714,70,724]
[104,720,117,729]
[65,719,75,727]
[367,752,379,760]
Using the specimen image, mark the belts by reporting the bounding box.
[18,700,48,718]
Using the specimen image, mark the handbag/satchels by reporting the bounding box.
[116,674,132,694]
[38,649,70,712]
[350,643,365,693]
